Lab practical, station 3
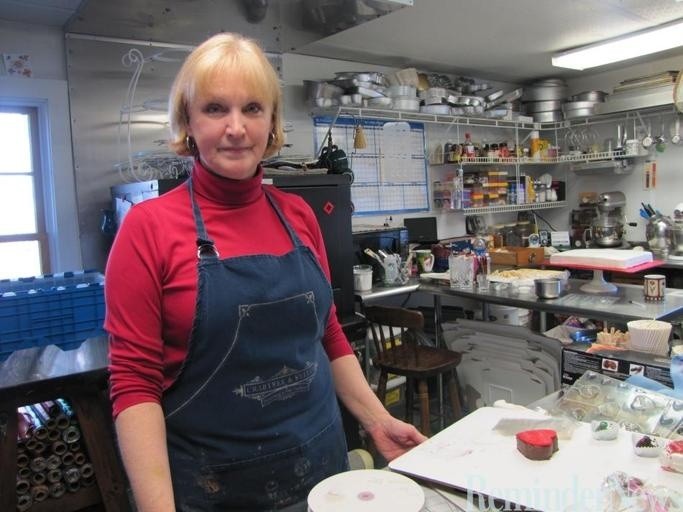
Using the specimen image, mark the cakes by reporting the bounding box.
[591,420,682,474]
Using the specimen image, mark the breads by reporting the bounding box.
[517,429,559,460]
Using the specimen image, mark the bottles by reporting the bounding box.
[444,131,540,162]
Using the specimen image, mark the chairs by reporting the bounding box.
[363,300,464,461]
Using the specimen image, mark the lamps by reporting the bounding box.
[344,111,367,150]
[550,17,682,71]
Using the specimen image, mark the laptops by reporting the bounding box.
[403,217,440,244]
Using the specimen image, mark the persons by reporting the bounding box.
[101,32,431,512]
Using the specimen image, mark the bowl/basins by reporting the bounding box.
[533,278,562,299]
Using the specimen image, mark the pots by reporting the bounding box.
[592,220,623,247]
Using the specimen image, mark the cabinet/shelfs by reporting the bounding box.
[312,100,683,217]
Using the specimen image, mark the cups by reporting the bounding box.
[412,249,434,273]
[352,264,373,291]
[381,253,412,285]
[448,253,473,289]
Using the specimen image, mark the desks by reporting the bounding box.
[0,337,133,511]
[351,240,683,417]
